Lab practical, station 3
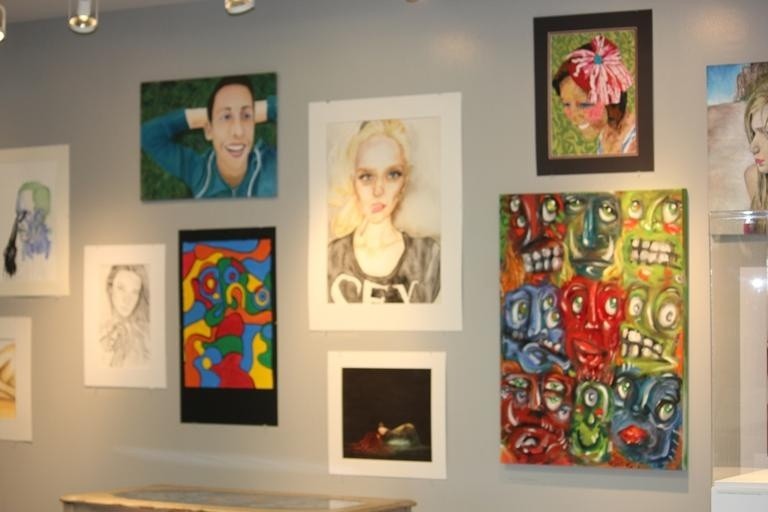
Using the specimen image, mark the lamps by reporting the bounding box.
[0,5,8,40]
[224,0,255,16]
[68,1,99,35]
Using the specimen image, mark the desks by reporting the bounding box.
[62,484,415,512]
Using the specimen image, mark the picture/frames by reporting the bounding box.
[532,9,655,177]
[327,349,446,481]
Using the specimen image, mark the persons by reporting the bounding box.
[328,120,440,304]
[98,265,150,369]
[552,34,637,157]
[733,62,768,234]
[140,76,277,199]
